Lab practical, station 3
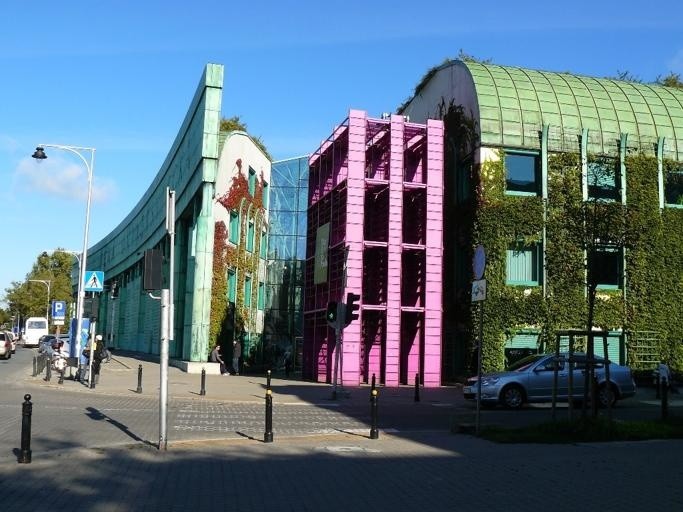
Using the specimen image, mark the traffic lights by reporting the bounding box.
[327,301,338,322]
[346,293,361,323]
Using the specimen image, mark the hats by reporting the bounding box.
[96,335,102,340]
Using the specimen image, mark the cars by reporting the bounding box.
[0,329,16,358]
[39,335,77,361]
[462,352,637,411]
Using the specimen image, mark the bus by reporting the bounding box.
[23,316,49,346]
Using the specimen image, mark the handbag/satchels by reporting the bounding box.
[98,348,108,359]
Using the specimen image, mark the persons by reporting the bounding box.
[232,338,241,376]
[93,333,106,385]
[652,360,669,400]
[282,347,292,378]
[208,343,230,376]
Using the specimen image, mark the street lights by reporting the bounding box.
[31,144,96,357]
